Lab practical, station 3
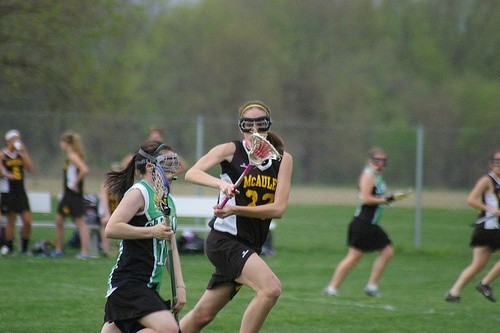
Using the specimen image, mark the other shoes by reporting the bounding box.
[325,285,337,296]
[362,286,379,296]
[475,281,497,302]
[77,253,88,260]
[49,249,65,257]
[445,293,460,302]
[21,249,33,256]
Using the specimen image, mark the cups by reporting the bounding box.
[14,142,22,151]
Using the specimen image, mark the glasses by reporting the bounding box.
[371,155,388,167]
[238,116,273,134]
[491,159,500,166]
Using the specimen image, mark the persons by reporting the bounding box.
[178,101,293,333]
[120,128,188,184]
[100,141,187,333]
[47,131,90,260]
[322,149,393,297]
[0,129,33,255]
[443,151,500,302]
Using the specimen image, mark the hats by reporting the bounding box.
[5,129,20,142]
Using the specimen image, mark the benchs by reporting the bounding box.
[174,197,277,251]
[3,193,102,258]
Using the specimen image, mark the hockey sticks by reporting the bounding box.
[385,190,411,203]
[153,164,183,333]
[206,133,280,230]
